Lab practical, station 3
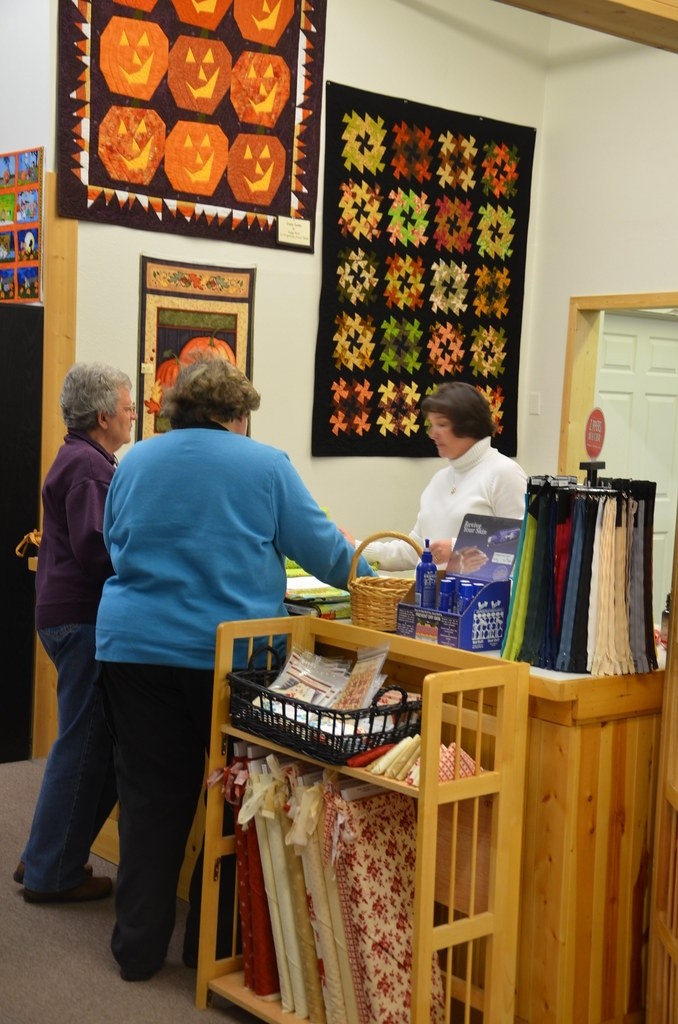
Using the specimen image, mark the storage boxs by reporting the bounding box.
[396,570,511,652]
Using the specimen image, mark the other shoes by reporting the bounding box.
[24,876,112,904]
[13,864,94,884]
[120,967,153,981]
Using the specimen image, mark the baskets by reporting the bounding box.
[225,646,423,766]
[346,531,423,632]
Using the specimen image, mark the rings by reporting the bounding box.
[435,558,438,561]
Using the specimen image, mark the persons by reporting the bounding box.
[93,359,379,982]
[13,362,140,904]
[338,382,528,573]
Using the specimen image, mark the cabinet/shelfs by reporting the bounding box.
[196,616,530,1024]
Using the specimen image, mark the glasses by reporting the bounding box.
[116,402,136,415]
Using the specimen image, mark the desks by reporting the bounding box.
[314,645,667,1024]
